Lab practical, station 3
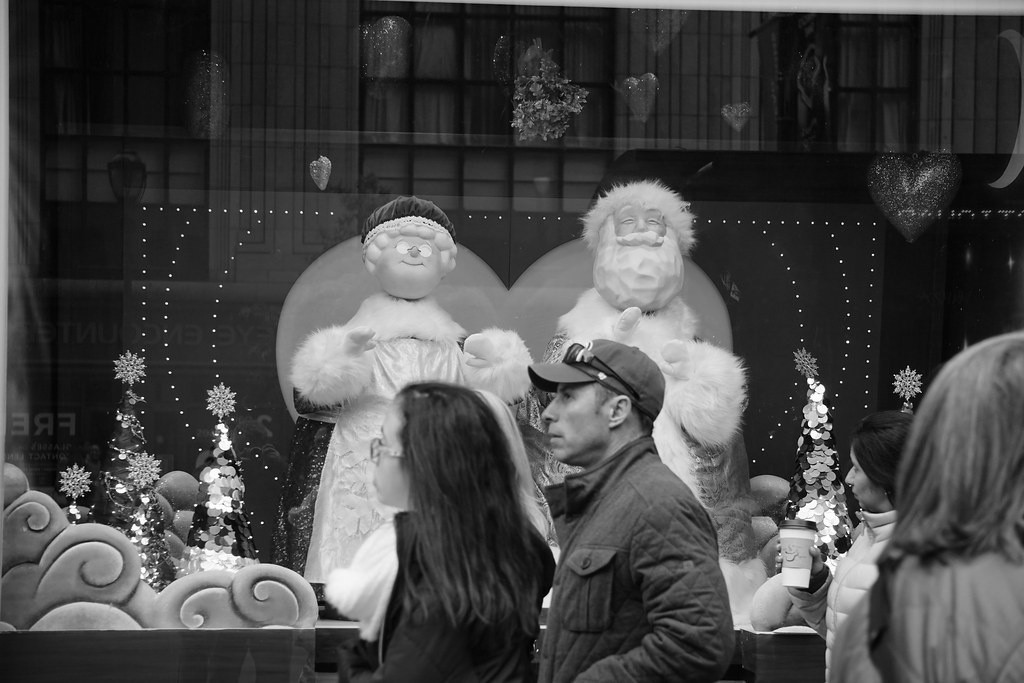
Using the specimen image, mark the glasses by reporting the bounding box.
[563,343,642,401]
[370,438,404,465]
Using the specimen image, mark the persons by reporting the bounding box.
[290,195,535,601]
[522,150,747,540]
[866,330,1024,683]
[527,340,736,683]
[339,381,557,683]
[775,411,916,683]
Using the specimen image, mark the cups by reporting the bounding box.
[778,520,817,588]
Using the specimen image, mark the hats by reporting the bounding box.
[527,339,666,418]
